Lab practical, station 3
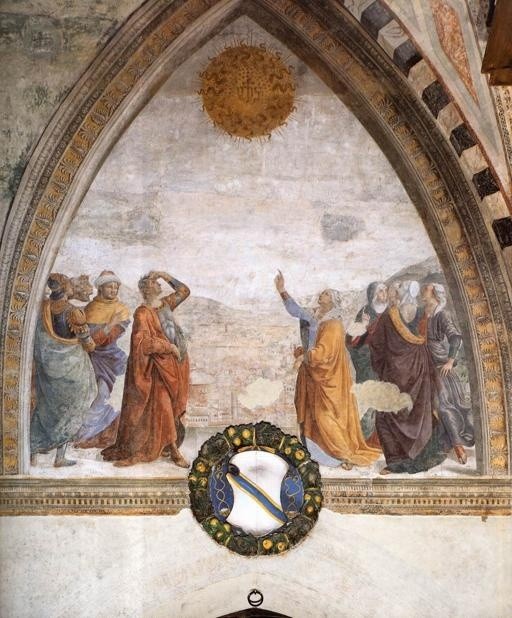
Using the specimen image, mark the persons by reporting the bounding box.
[30,269,195,466]
